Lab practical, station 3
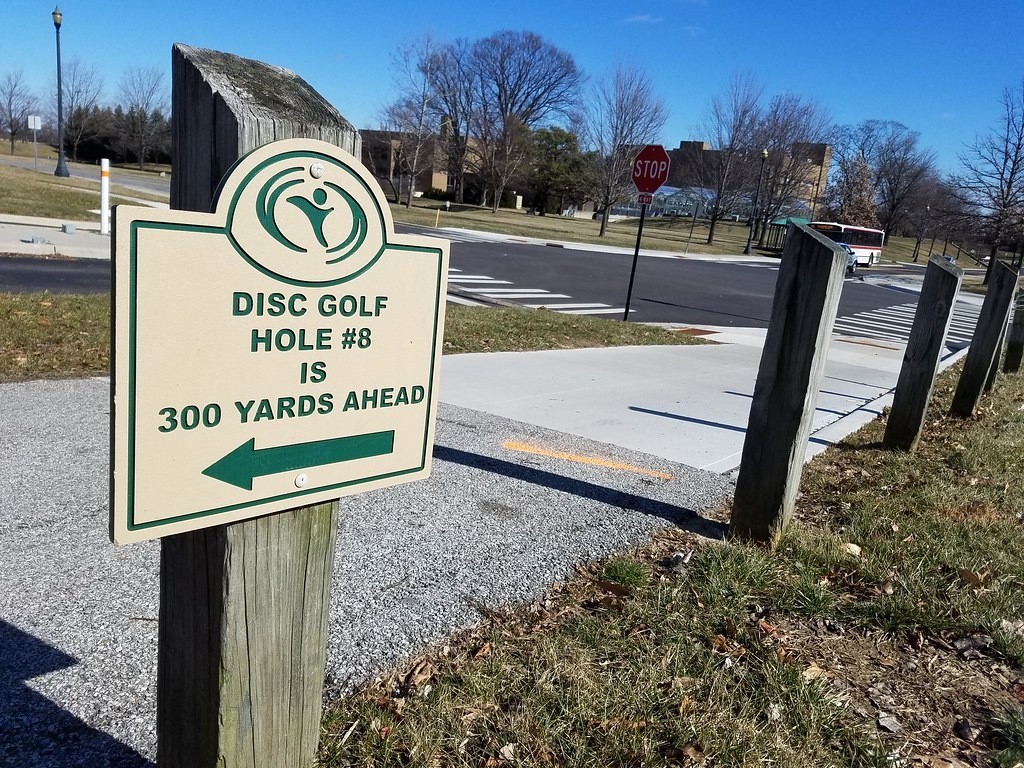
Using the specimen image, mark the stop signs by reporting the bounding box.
[631,144,671,195]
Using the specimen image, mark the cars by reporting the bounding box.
[835,240,859,274]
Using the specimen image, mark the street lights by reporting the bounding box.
[913,205,929,262]
[743,149,768,256]
[51,5,72,176]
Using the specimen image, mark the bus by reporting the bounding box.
[802,221,886,268]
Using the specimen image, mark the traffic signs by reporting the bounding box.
[108,205,459,548]
